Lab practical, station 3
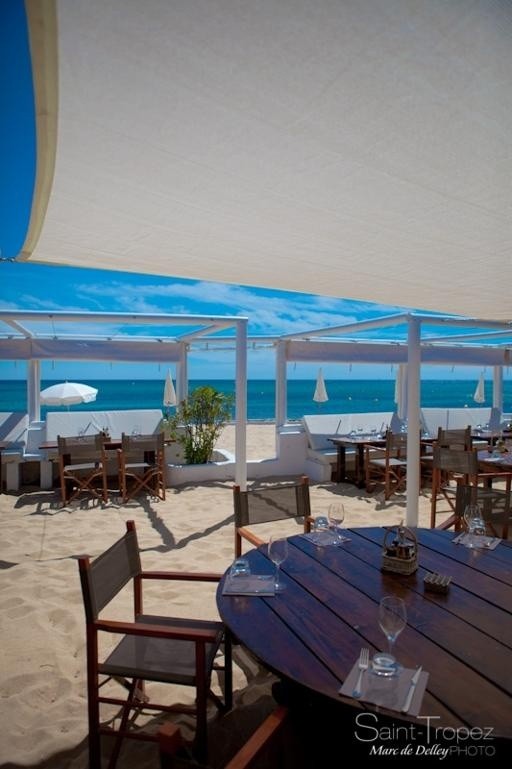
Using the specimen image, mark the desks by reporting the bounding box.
[0,441,11,449]
[39,438,176,499]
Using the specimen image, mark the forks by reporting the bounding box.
[352,647,369,698]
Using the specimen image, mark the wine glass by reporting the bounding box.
[328,503,344,545]
[464,504,481,549]
[268,533,289,593]
[377,597,408,655]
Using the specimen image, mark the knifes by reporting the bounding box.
[401,666,422,713]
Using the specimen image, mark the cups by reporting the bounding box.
[367,653,400,699]
[470,518,486,543]
[312,516,329,540]
[228,560,251,590]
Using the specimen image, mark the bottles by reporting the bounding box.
[386,530,415,560]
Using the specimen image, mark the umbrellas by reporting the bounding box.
[40,380,98,410]
[162,367,177,415]
[474,372,486,407]
[312,367,329,414]
